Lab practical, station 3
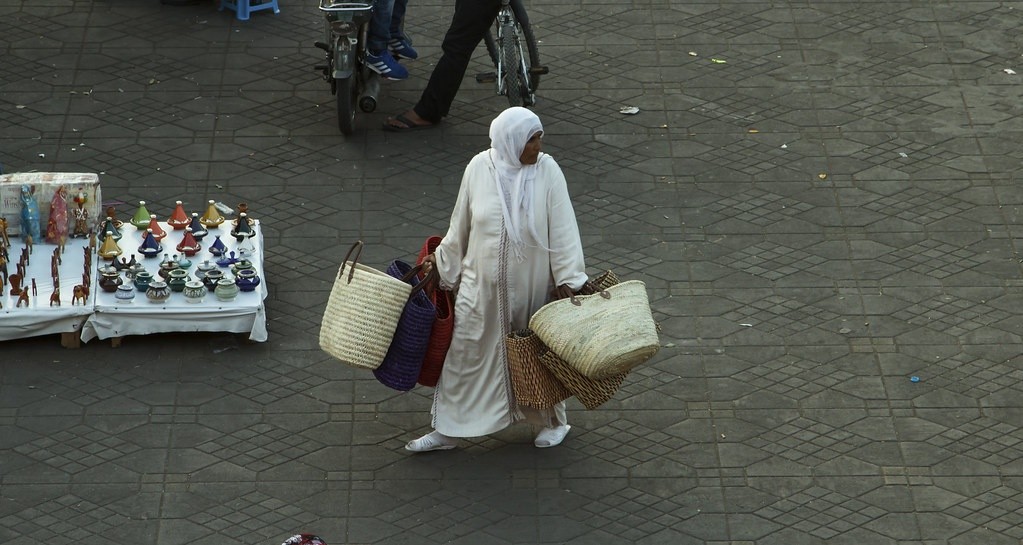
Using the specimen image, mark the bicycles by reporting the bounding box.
[475,0,549,108]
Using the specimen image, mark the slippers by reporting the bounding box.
[535,424,571,447]
[381,110,435,129]
[405,433,458,451]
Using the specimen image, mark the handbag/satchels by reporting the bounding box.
[320,235,456,392]
[504,270,664,411]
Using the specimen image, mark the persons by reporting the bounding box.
[358,0,418,80]
[383,0,504,132]
[405,107,588,452]
[72,192,89,239]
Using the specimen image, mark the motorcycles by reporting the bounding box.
[315,0,399,136]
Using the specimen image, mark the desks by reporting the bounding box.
[1,219,262,349]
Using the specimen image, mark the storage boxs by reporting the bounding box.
[0,172,102,236]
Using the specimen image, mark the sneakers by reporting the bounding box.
[358,49,410,81]
[386,37,418,60]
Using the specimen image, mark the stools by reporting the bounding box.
[217,0,280,20]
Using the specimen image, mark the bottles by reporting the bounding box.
[97,200,260,304]
